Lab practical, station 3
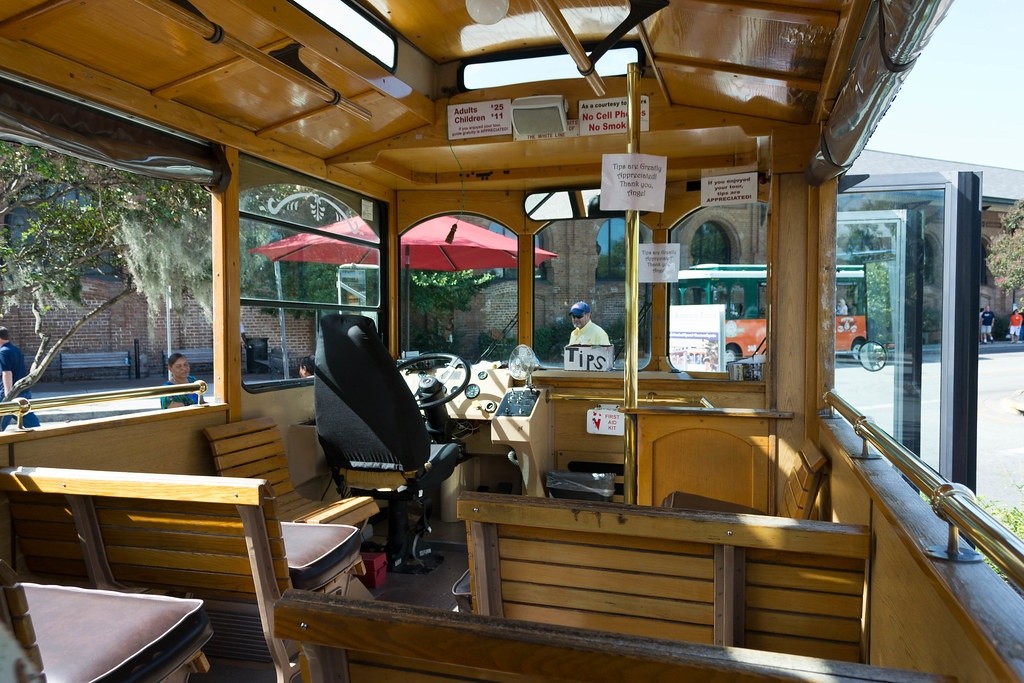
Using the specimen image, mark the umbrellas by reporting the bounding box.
[247,216,558,352]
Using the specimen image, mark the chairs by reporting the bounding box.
[313,312,461,574]
[203,416,381,525]
[662,438,830,523]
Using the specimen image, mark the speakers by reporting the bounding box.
[510,95,568,135]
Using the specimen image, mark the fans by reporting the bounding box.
[508,344,537,388]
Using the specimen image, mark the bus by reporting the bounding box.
[668,263,868,374]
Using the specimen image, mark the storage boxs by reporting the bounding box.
[355,550,387,591]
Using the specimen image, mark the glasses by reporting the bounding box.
[571,314,589,319]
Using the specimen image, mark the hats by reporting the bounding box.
[568,300,591,316]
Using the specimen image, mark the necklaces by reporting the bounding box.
[171,377,178,383]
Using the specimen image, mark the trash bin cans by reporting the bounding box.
[245,337,270,373]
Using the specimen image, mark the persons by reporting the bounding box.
[160,353,198,409]
[299,357,314,378]
[569,301,610,345]
[1007,310,1024,344]
[979,305,995,344]
[0,326,40,431]
[837,299,848,316]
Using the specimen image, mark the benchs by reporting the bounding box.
[1,467,964,683]
[162,347,214,377]
[60,350,132,382]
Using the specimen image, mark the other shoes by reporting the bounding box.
[990,338,993,344]
[981,341,987,345]
